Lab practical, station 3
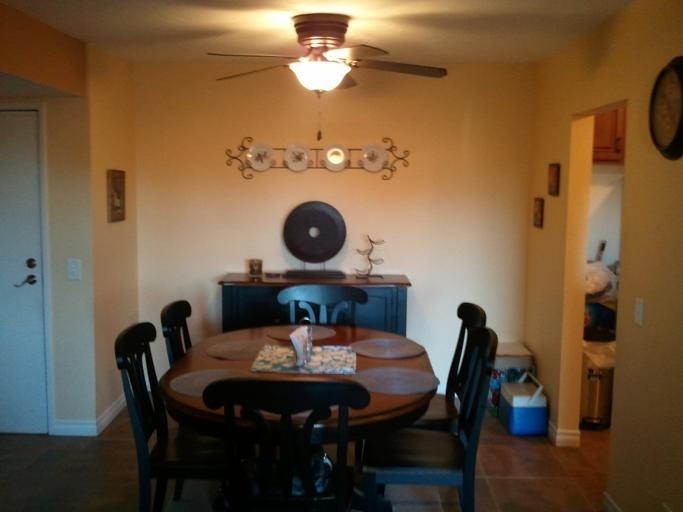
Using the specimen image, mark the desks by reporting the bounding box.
[168,323,441,512]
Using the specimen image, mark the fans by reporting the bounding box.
[205,13,448,91]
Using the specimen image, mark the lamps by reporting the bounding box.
[286,57,351,94]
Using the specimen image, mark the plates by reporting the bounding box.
[283,144,310,172]
[245,143,275,172]
[361,144,386,172]
[322,143,351,171]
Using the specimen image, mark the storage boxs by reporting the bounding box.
[497,371,552,437]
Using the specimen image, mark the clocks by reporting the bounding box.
[649,55,683,158]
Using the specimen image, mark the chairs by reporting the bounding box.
[161,299,192,368]
[362,324,498,509]
[393,301,488,431]
[113,320,241,511]
[199,370,373,512]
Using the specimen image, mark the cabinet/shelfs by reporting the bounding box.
[218,271,411,338]
[591,105,624,164]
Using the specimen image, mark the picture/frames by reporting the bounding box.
[107,170,125,221]
[548,163,560,194]
[533,197,543,227]
